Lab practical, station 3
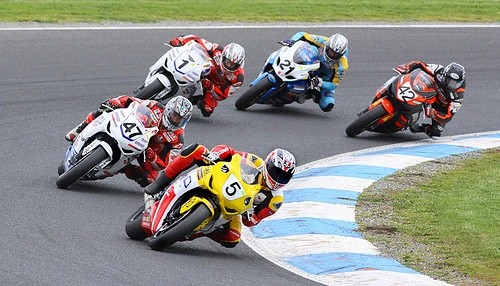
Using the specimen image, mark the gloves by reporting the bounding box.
[203,152,219,164]
[396,64,409,74]
[170,39,181,46]
[145,147,156,162]
[283,40,295,47]
[313,75,323,87]
[202,78,211,89]
[427,108,437,119]
[109,97,122,108]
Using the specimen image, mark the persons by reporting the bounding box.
[64,96,192,188]
[249,31,349,113]
[144,143,297,249]
[169,34,245,118]
[376,61,466,139]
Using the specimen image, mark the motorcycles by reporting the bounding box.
[235,40,326,111]
[346,61,446,138]
[56,101,161,189]
[124,154,264,251]
[133,42,213,105]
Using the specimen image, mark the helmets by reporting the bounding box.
[162,96,193,132]
[441,62,465,93]
[220,43,245,75]
[324,34,348,63]
[262,149,296,191]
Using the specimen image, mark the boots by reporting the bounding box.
[65,112,94,142]
[144,170,171,196]
